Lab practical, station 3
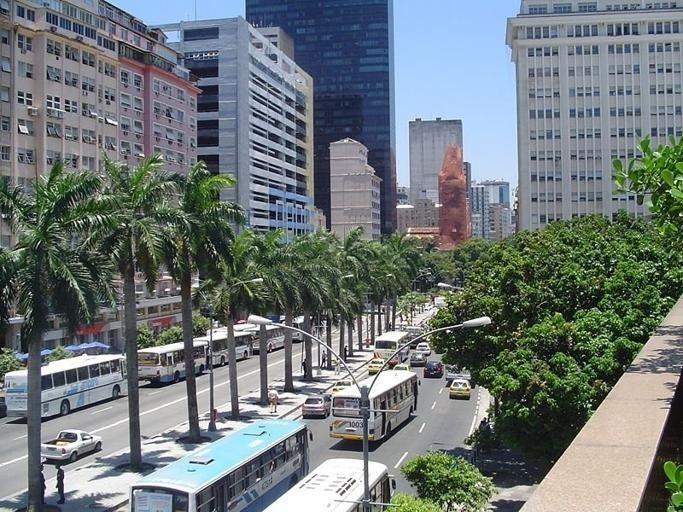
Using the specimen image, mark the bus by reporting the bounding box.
[259,458,398,511]
[374,331,410,363]
[3,352,124,420]
[329,369,422,443]
[136,314,312,384]
[446,364,473,380]
[131,417,313,512]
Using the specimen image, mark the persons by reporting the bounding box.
[320,350,327,367]
[41,464,47,504]
[55,463,65,504]
[302,359,307,378]
[267,386,279,414]
[343,345,348,361]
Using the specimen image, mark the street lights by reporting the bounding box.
[191,278,263,432]
[411,273,431,321]
[318,274,354,375]
[366,273,393,347]
[438,282,463,290]
[247,314,492,511]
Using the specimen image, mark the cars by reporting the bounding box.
[424,361,445,378]
[449,379,471,400]
[411,334,431,366]
[369,358,389,374]
[302,395,332,418]
[394,363,410,371]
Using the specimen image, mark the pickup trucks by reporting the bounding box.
[41,429,102,460]
